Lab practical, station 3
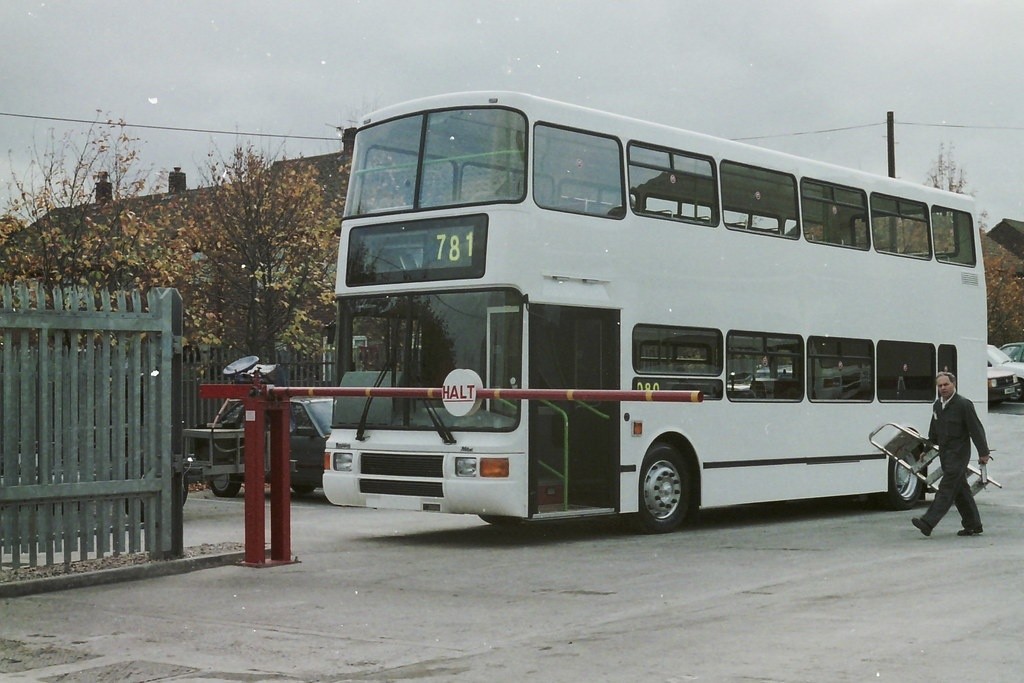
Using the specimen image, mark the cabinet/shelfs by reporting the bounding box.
[182,428,245,476]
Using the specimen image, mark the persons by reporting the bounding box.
[911,372,990,535]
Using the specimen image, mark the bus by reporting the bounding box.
[322,88,987,536]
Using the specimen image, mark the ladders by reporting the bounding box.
[868,422,1003,504]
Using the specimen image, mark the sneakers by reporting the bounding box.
[911,516,933,537]
[957,525,983,537]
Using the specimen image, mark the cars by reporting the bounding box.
[989,341,1024,408]
[190,395,334,498]
[727,354,871,401]
